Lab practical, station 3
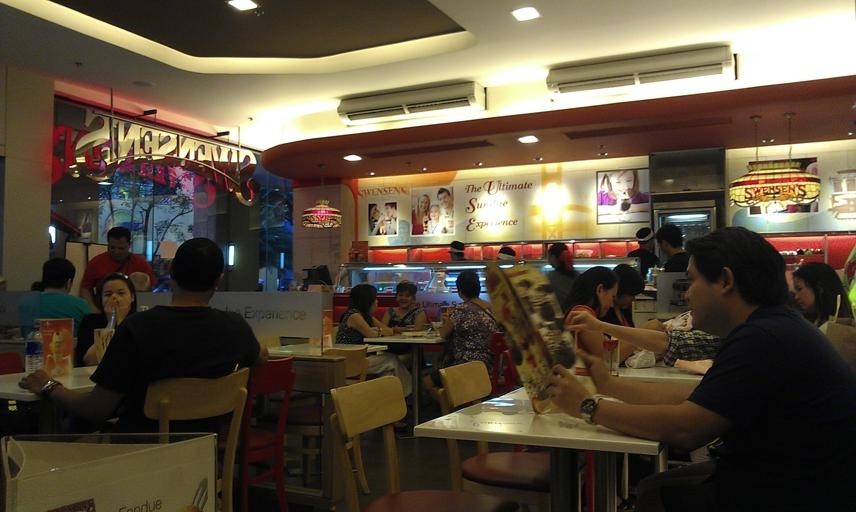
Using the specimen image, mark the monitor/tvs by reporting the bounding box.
[654,207,717,258]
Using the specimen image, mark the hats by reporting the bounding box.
[636,227,654,243]
[447,241,465,254]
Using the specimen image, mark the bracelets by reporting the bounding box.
[39,379,60,397]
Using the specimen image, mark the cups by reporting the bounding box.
[600,335,619,376]
[432,322,443,336]
[94,328,115,364]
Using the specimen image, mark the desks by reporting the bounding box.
[568,355,704,380]
[1,366,114,437]
[411,376,706,510]
[363,330,448,441]
[261,341,390,483]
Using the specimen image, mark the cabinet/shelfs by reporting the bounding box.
[331,255,641,328]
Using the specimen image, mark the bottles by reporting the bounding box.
[106,311,118,329]
[25,324,44,375]
[646,264,665,288]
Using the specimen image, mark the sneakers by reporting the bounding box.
[396,426,416,439]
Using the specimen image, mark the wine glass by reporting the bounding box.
[49,342,66,376]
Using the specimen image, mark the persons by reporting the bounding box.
[380,280,427,368]
[334,284,416,439]
[74,272,137,368]
[420,271,496,388]
[20,257,94,338]
[16,237,261,432]
[540,222,855,511]
[598,170,649,206]
[369,202,397,235]
[449,241,466,261]
[412,188,454,233]
[80,227,158,313]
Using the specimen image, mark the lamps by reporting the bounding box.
[302,164,343,229]
[729,112,822,208]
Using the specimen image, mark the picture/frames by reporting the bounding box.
[596,167,651,224]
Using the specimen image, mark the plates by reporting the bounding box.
[401,331,431,338]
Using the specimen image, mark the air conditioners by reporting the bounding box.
[336,77,487,124]
[546,40,737,95]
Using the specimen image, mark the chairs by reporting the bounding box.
[140,365,249,507]
[325,343,369,389]
[239,353,296,508]
[325,374,503,512]
[438,358,560,496]
